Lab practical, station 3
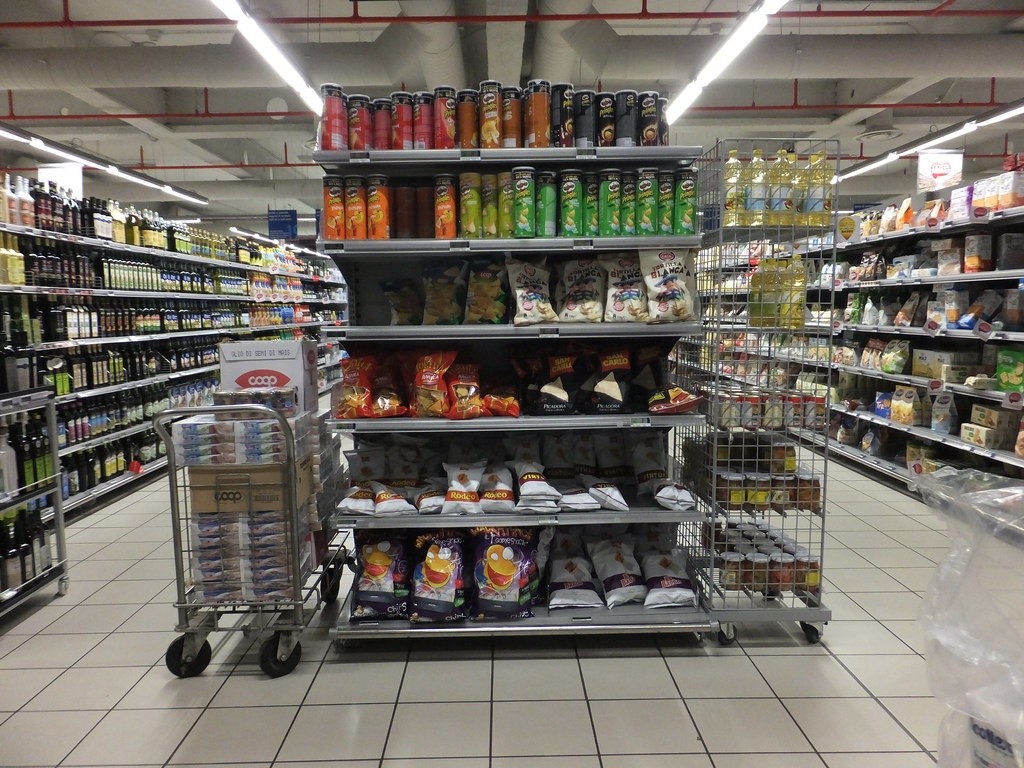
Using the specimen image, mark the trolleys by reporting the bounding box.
[154,403,350,678]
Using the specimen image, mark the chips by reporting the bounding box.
[336,384,519,414]
[392,281,507,323]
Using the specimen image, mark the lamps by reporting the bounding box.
[0,122,209,206]
[829,99,1023,186]
[229,225,331,259]
[666,1,794,127]
[211,1,323,123]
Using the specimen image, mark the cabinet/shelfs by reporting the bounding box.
[0,223,345,536]
[313,144,722,651]
[674,172,1023,538]
[674,137,844,643]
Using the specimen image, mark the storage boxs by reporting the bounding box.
[170,338,345,603]
[695,173,1022,477]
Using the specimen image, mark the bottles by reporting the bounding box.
[744,256,808,329]
[695,147,833,234]
[2,172,344,300]
[0,293,351,505]
[0,504,52,595]
